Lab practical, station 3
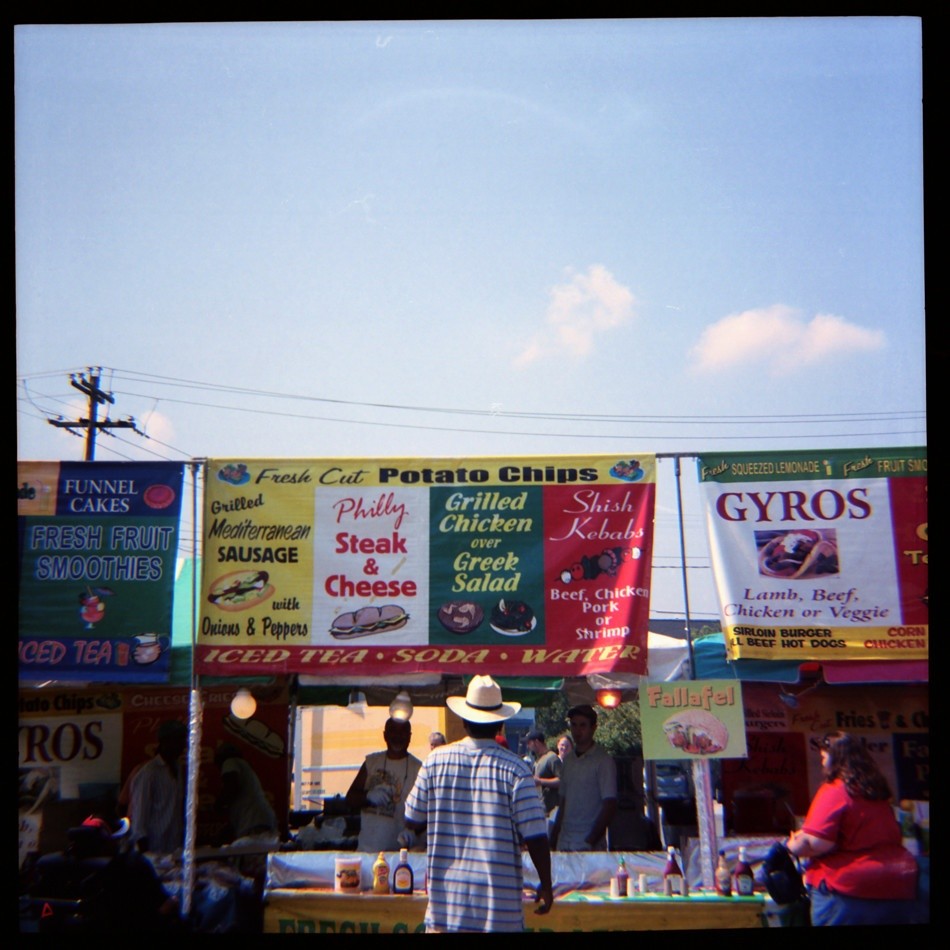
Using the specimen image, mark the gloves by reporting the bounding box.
[374,784,397,795]
[365,790,390,807]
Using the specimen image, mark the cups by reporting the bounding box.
[335,857,362,894]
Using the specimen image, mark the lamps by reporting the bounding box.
[230,686,257,719]
[347,689,414,723]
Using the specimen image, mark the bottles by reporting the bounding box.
[372,850,389,894]
[663,846,688,898]
[393,848,412,894]
[609,855,647,899]
[734,846,754,895]
[714,850,731,896]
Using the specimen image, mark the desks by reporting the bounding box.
[262,890,788,932]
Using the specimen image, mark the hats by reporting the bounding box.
[446,675,521,724]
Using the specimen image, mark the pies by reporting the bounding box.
[663,708,728,755]
[758,531,836,578]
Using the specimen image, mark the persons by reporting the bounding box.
[787,728,921,925]
[523,702,621,852]
[126,718,189,851]
[399,673,554,934]
[344,718,429,852]
[211,742,280,839]
[429,732,446,751]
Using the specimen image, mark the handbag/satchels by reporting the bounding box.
[762,844,800,899]
